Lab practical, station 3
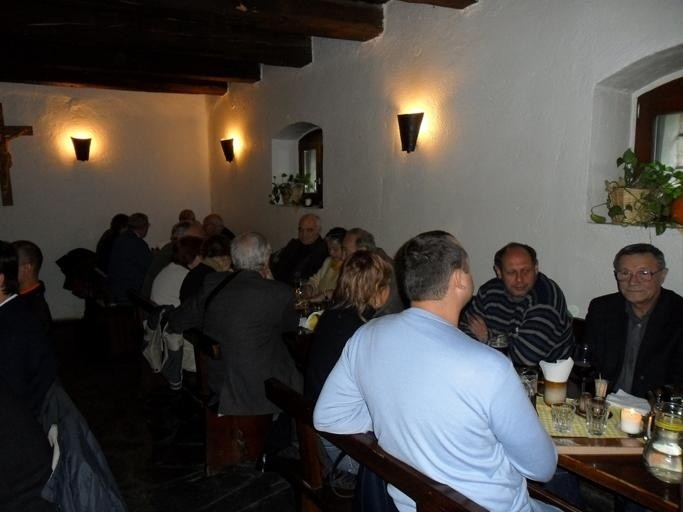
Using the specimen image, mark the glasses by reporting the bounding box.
[613,266,665,281]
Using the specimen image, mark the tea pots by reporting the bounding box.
[642,401,683,485]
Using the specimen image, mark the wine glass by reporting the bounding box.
[487,335,514,371]
[572,342,594,405]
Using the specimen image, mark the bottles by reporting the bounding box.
[592,396,605,415]
[584,399,610,435]
[551,403,576,433]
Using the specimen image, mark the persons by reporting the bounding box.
[301,227,348,303]
[202,214,237,242]
[91,213,129,263]
[342,228,405,315]
[150,235,201,308]
[195,230,300,417]
[458,241,575,376]
[140,223,184,299]
[266,213,330,286]
[106,213,150,305]
[302,250,395,476]
[312,231,565,512]
[574,244,683,404]
[180,243,232,306]
[11,240,53,338]
[1,241,61,511]
[179,209,196,222]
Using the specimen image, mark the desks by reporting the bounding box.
[558,454,683,512]
[281,302,330,366]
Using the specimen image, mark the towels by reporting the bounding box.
[606,389,651,416]
[539,357,574,383]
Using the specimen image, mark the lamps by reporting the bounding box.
[220,138,237,162]
[70,137,94,161]
[398,112,423,152]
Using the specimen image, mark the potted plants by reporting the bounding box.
[269,171,315,204]
[590,147,683,236]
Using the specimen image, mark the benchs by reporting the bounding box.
[264,376,583,512]
[572,316,587,363]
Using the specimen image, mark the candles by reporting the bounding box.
[621,408,642,434]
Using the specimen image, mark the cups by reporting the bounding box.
[518,367,538,411]
[543,380,567,406]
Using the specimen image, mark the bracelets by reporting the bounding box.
[323,289,329,300]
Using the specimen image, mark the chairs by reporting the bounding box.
[126,288,263,477]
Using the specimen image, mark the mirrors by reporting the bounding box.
[635,76,683,226]
[298,128,322,207]
